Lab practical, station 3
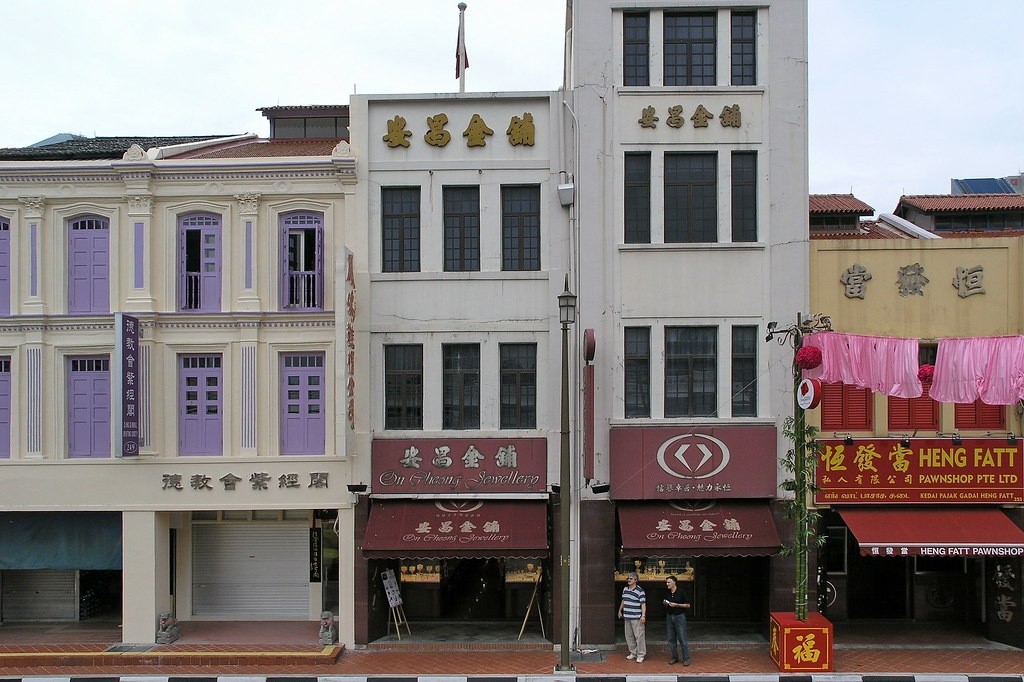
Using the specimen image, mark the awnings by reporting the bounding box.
[836,508,1024,556]
[617,501,781,558]
[362,501,547,559]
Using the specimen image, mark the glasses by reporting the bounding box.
[626,578,635,581]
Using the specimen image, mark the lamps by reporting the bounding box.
[936,431,961,446]
[834,431,853,445]
[887,432,909,447]
[987,431,1017,445]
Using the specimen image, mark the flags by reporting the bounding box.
[456,25,468,78]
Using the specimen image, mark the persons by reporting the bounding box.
[618,572,646,662]
[663,576,690,666]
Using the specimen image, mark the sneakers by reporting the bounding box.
[683,658,692,666]
[669,657,679,663]
[625,652,637,659]
[635,656,646,663]
